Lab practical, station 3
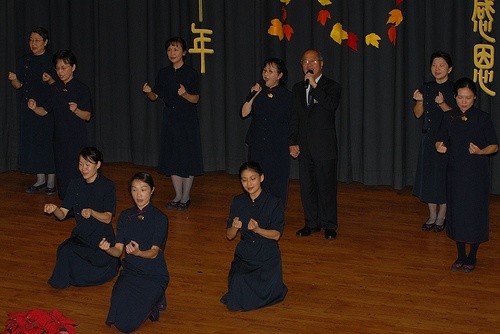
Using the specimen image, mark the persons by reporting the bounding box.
[435,78,500,273]
[44,148,121,288]
[27,49,94,221]
[290,49,342,240]
[143,36,204,211]
[8,26,57,195]
[219,161,288,312]
[413,51,456,232]
[242,59,291,210]
[98,173,170,334]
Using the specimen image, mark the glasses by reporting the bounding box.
[303,59,320,64]
[28,39,44,43]
[54,67,69,71]
[263,69,278,74]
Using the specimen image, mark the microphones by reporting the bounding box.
[305,69,314,89]
[246,78,267,103]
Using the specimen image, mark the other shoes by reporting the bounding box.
[46,183,56,194]
[463,256,477,272]
[167,199,181,208]
[177,198,191,209]
[422,214,435,232]
[451,256,463,271]
[434,215,447,232]
[160,294,168,311]
[26,182,46,193]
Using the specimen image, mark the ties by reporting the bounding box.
[307,83,315,105]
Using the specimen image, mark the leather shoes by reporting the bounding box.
[325,228,338,240]
[295,226,321,236]
